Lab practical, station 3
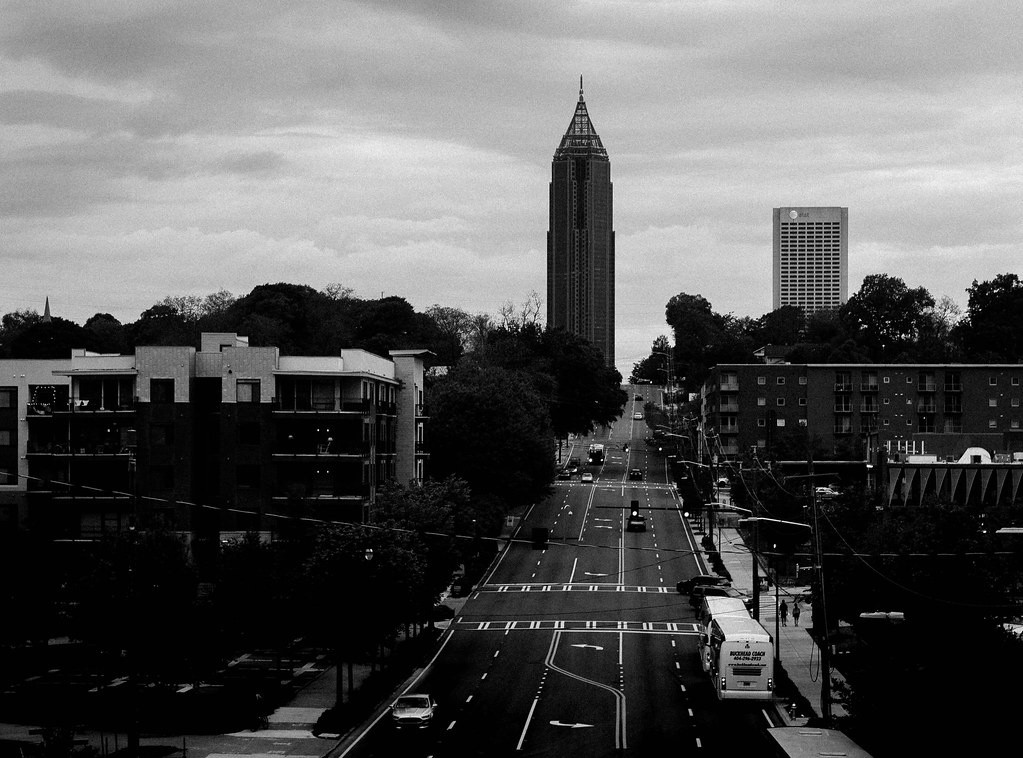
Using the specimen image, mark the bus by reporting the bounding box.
[763,725,873,758]
[588,443,605,465]
[697,596,774,704]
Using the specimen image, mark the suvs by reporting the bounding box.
[676,574,733,595]
[689,583,754,611]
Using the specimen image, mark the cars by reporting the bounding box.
[626,514,648,532]
[389,693,437,730]
[580,472,594,484]
[629,468,643,481]
[634,393,644,401]
[633,412,643,420]
[559,457,580,480]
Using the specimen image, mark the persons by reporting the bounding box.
[779,600,788,627]
[718,516,730,527]
[792,603,800,627]
